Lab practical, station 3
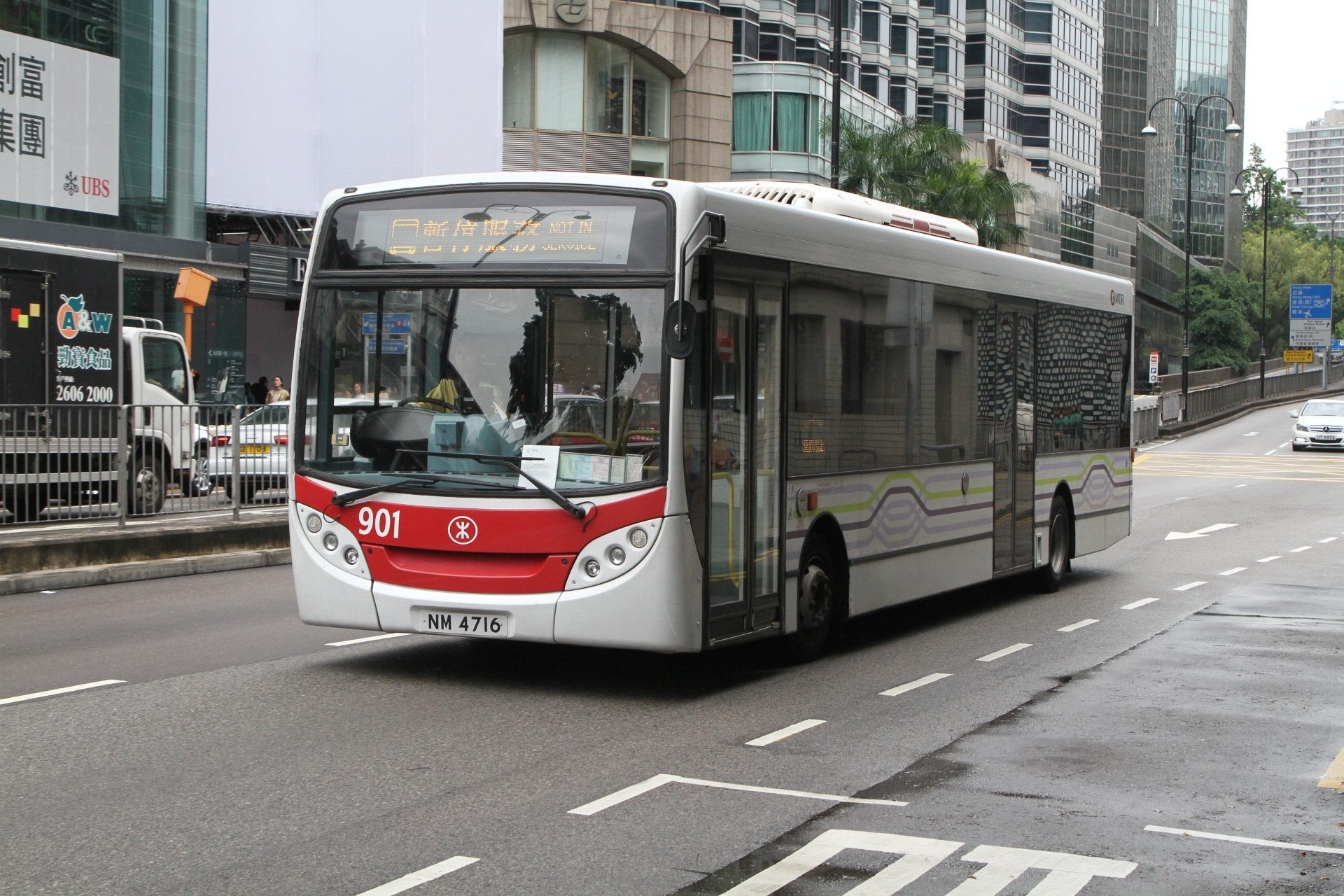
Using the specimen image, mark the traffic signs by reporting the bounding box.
[1282,349,1314,364]
[1289,318,1332,348]
[1288,284,1334,319]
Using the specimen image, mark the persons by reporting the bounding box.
[169,360,420,415]
[1052,404,1086,449]
[400,332,505,415]
[579,377,657,403]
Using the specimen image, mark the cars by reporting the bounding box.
[206,397,419,504]
[1330,350,1343,363]
[177,424,214,497]
[1291,399,1344,451]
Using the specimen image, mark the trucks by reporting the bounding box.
[0,234,197,524]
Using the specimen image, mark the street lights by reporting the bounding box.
[1139,96,1242,432]
[1229,166,1305,399]
[1297,203,1344,335]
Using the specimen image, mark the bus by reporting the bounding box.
[284,167,1142,667]
